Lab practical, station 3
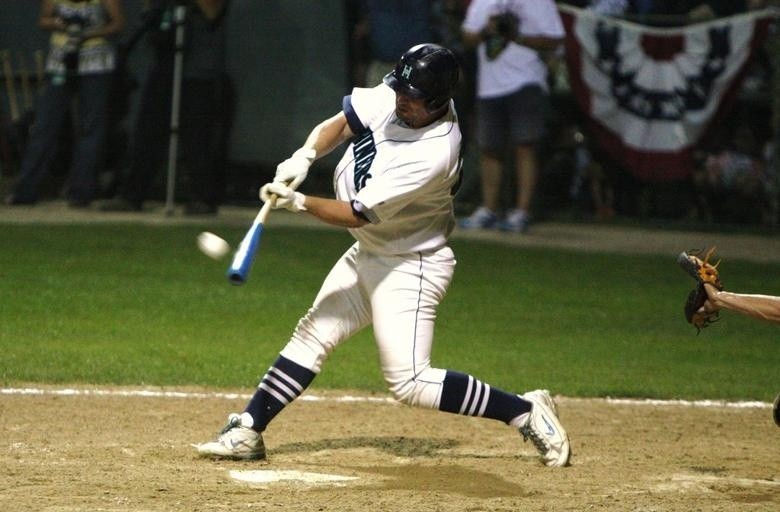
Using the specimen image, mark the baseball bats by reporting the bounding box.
[226,182,290,287]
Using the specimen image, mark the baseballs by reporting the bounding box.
[195,231,231,260]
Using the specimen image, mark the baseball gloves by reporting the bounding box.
[677,246,724,336]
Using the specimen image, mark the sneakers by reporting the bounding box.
[459,206,532,232]
[3,188,141,212]
[519,388,570,467]
[198,412,265,460]
[182,201,218,218]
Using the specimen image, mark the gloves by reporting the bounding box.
[272,146,317,191]
[259,181,306,214]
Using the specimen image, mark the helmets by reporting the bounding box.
[382,43,460,115]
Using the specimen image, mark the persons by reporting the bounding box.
[110,2,234,217]
[457,2,568,233]
[5,1,125,208]
[678,250,780,426]
[191,40,569,466]
